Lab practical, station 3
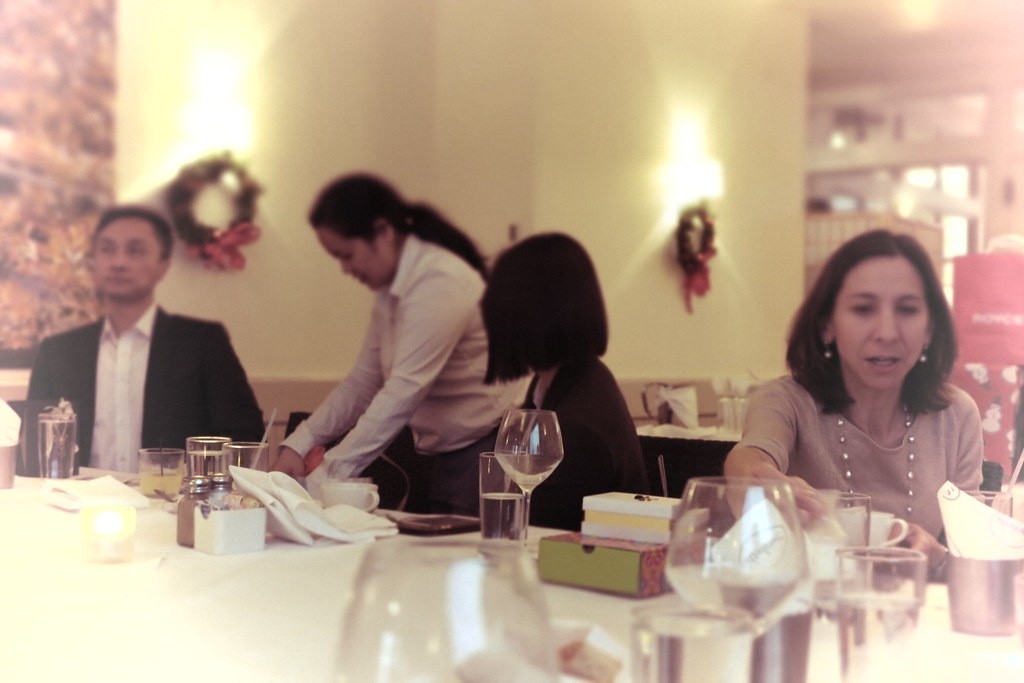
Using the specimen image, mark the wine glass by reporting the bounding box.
[494,409,564,559]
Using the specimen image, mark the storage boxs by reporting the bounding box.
[535,531,671,601]
[582,491,682,547]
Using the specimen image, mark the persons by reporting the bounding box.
[16,198,266,478]
[724,230,986,583]
[479,230,654,532]
[269,169,536,516]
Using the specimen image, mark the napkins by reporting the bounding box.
[662,386,699,430]
[936,479,1021,560]
[43,474,148,513]
[230,464,400,548]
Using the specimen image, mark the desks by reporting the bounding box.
[0,473,1022,683]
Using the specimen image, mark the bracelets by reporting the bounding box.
[932,547,953,583]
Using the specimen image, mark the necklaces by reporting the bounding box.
[837,396,914,526]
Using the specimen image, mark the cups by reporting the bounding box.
[186,436,231,493]
[658,475,809,640]
[38,414,76,479]
[799,486,1023,683]
[223,441,269,494]
[479,452,530,540]
[334,535,558,682]
[138,447,185,501]
[320,481,380,513]
[631,602,769,683]
[718,396,747,435]
